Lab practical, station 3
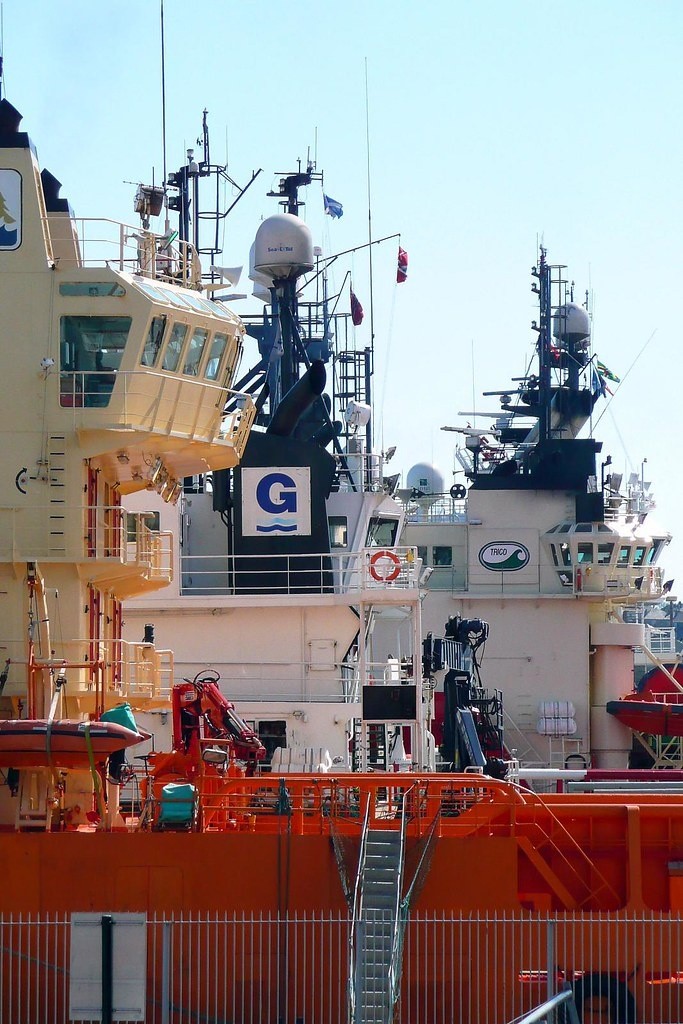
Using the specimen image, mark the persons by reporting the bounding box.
[561,974,637,1024]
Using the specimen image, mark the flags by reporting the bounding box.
[397,247,408,282]
[592,371,614,398]
[597,361,620,383]
[351,294,364,326]
[324,195,343,219]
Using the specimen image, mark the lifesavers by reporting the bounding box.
[370,551,401,582]
[565,754,586,771]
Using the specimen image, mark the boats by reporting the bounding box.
[0,0,683,1024]
[606,663,683,737]
